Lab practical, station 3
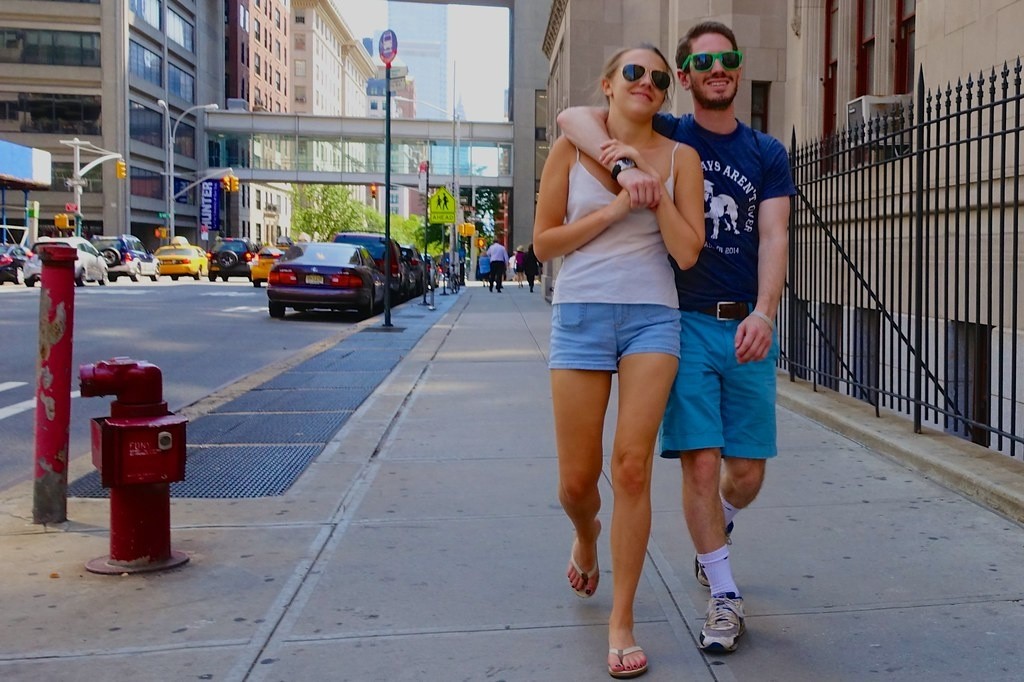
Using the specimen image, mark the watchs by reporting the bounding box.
[611,155,636,179]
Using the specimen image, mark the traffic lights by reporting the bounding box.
[117,160,126,178]
[222,176,231,192]
[231,176,239,191]
[154,226,166,238]
[371,183,376,198]
[474,238,486,247]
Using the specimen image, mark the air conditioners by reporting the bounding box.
[846,94,912,149]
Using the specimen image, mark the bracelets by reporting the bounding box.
[750,310,776,333]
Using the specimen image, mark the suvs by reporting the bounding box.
[89,234,160,282]
[333,230,440,301]
[207,238,259,282]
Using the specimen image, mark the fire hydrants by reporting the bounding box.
[78,357,190,575]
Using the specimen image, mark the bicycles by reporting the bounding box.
[449,264,460,294]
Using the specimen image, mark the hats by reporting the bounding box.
[517,245,524,251]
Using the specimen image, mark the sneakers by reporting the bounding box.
[700,592,746,653]
[695,520,735,588]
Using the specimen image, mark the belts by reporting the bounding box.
[693,299,749,321]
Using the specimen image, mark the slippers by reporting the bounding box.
[567,519,601,599]
[607,645,648,677]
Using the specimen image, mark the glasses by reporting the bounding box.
[682,51,743,73]
[608,64,670,90]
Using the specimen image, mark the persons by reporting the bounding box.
[485,238,507,293]
[523,243,543,293]
[479,244,506,289]
[532,41,704,678]
[556,18,796,652]
[508,251,517,273]
[419,161,427,172]
[514,244,526,288]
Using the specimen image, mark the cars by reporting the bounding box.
[0,244,30,285]
[154,236,208,280]
[22,237,109,287]
[267,243,393,318]
[251,244,293,287]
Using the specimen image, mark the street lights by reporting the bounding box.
[393,96,461,266]
[158,100,219,239]
